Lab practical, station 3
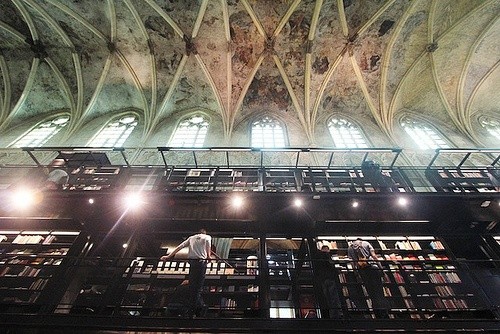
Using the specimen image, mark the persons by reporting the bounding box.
[160,226,212,319]
[348,238,392,320]
[313,245,343,319]
[361,158,389,192]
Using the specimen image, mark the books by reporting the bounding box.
[200,255,259,311]
[64,165,111,190]
[314,239,479,320]
[161,168,376,194]
[436,169,498,193]
[124,231,190,309]
[380,167,406,193]
[0,235,70,305]
